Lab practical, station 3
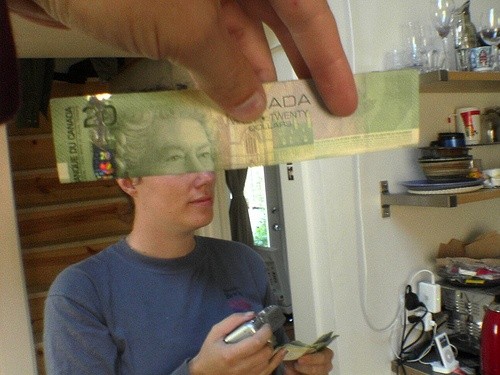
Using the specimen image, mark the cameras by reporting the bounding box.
[224,304,288,347]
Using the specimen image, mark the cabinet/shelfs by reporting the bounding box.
[380,70,500,208]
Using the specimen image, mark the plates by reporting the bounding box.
[399,146,486,195]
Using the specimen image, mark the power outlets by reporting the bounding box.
[401,294,414,325]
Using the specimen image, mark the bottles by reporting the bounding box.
[480,294,500,375]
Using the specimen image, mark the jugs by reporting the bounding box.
[455,1,477,72]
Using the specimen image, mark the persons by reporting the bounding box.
[43,172,333,375]
[0,0,359,124]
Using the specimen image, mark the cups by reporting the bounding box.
[448,106,482,145]
[470,46,499,72]
[399,20,428,68]
[383,47,404,70]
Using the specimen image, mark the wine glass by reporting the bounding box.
[431,0,456,71]
[477,6,500,73]
